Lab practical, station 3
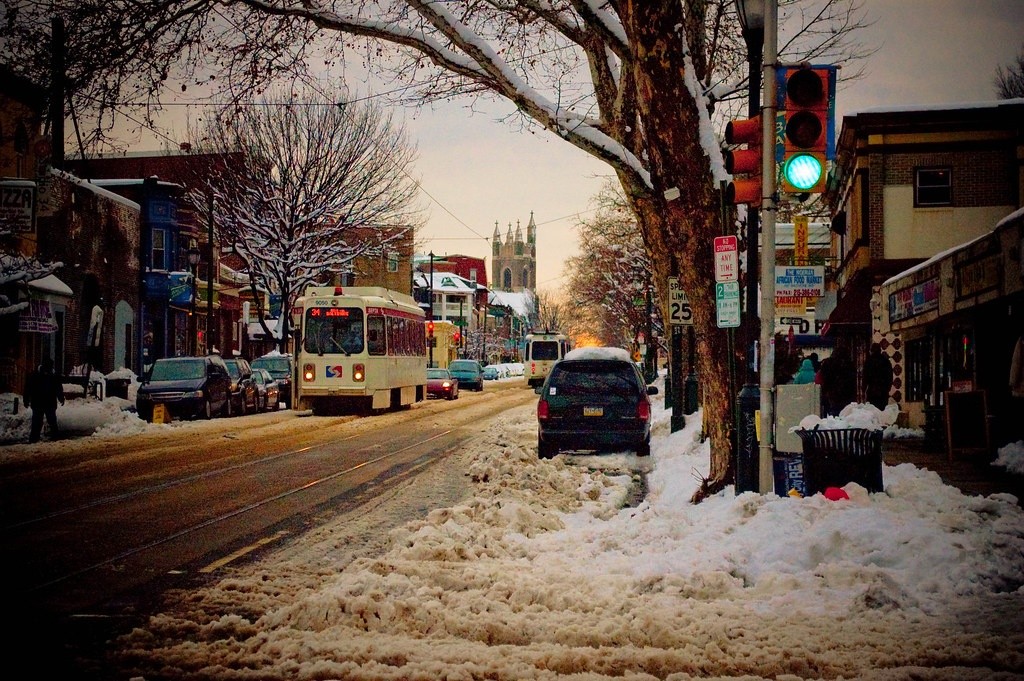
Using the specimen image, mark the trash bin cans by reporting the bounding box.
[103,378,131,400]
[793,426,888,498]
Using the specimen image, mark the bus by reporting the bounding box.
[524,331,571,388]
[297,284,427,415]
[425,320,466,371]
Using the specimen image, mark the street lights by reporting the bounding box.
[187,242,201,357]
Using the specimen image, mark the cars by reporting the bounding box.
[447,359,484,392]
[534,357,660,460]
[487,364,524,378]
[252,369,279,412]
[426,368,459,400]
[137,356,232,422]
[484,368,499,381]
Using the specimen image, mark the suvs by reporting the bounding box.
[223,357,259,415]
[251,355,292,410]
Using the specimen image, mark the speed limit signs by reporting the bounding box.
[668,276,694,324]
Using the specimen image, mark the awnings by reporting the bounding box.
[821,296,872,338]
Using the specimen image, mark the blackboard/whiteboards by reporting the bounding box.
[944,390,990,451]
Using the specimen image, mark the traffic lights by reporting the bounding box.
[724,115,763,208]
[455,333,459,345]
[781,68,829,194]
[428,322,434,337]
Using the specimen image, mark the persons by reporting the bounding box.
[793,352,850,419]
[23,358,67,443]
[319,322,340,353]
[861,343,893,411]
